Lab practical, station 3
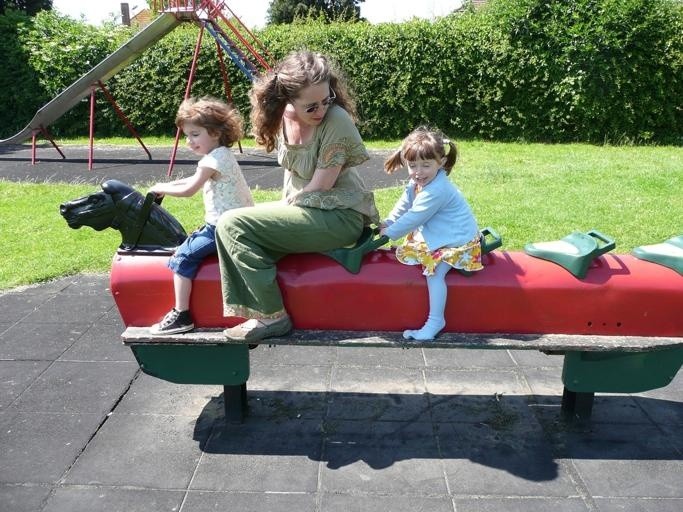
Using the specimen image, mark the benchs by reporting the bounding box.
[109,246,683,422]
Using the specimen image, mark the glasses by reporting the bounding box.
[286,86,338,118]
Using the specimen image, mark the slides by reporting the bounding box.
[0,14,182,151]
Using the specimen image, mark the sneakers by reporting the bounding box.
[148,307,195,335]
[222,314,294,343]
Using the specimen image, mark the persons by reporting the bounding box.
[376,123,485,340]
[147,93,262,336]
[212,50,379,344]
